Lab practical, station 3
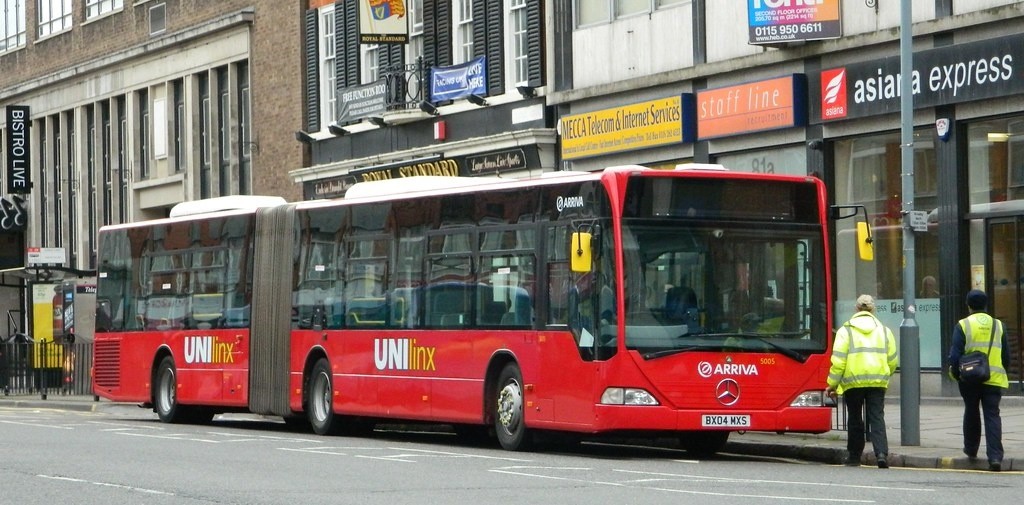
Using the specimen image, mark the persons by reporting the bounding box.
[826,294,897,468]
[722,312,768,352]
[948,290,1010,467]
[920,275,940,298]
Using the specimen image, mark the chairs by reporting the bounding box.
[665,287,700,332]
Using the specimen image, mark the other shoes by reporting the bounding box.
[987,455,1001,472]
[876,452,889,468]
[841,455,861,466]
[962,446,977,458]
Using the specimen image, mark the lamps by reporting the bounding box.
[465,94,490,107]
[419,100,440,117]
[516,86,537,100]
[327,125,350,137]
[367,116,389,129]
[295,131,316,144]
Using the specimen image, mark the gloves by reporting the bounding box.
[949,366,958,383]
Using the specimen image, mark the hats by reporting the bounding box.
[966,289,987,309]
[738,312,763,325]
[856,294,874,310]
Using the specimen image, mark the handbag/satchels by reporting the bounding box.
[956,349,990,388]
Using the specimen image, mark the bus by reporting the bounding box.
[88,161,875,458]
